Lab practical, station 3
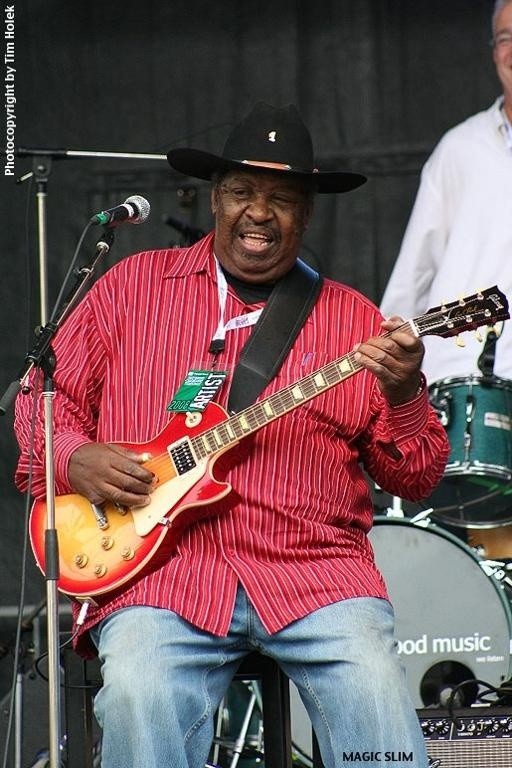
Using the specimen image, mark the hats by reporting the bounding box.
[167,106,367,193]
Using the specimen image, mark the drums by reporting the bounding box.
[244,513,512,768]
[209,680,266,768]
[420,374,512,535]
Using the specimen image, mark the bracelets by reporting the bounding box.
[417,376,424,395]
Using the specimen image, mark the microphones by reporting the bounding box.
[95,195,150,226]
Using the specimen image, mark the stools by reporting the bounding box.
[63,638,293,767]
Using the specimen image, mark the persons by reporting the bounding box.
[12,108,455,768]
[380,3,510,388]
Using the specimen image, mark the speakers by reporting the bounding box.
[415,705,512,768]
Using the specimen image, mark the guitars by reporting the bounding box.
[28,284,510,601]
